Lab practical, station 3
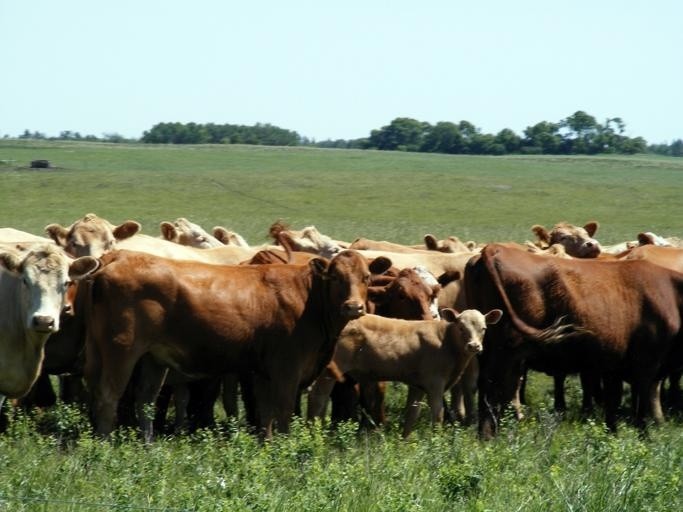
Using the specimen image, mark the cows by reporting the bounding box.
[0,215,683,453]
